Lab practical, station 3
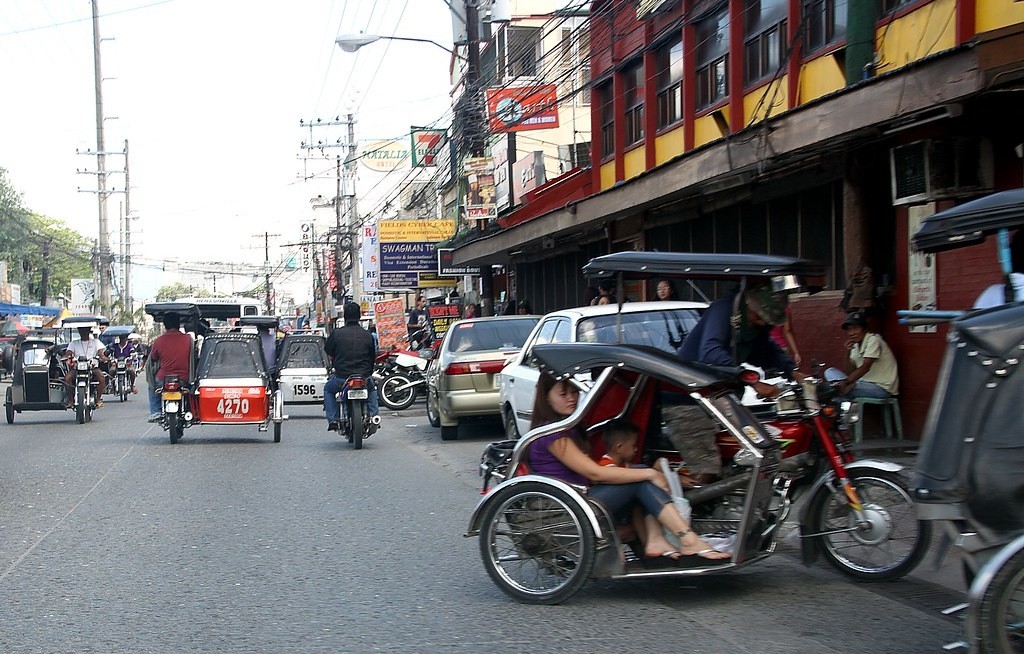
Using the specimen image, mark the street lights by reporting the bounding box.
[335,30,494,316]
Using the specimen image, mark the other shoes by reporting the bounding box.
[110,387,115,393]
[95,401,103,408]
[329,422,338,431]
[66,401,75,408]
[148,412,162,422]
[131,385,138,394]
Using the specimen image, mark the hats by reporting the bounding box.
[840,311,869,329]
[743,279,788,327]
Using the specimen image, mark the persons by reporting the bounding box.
[64,325,151,409]
[147,312,275,423]
[527,372,733,568]
[369,295,528,351]
[591,279,900,484]
[323,302,381,432]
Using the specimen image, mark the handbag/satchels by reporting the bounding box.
[659,458,691,548]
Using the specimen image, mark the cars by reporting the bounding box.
[419,314,544,441]
[500,300,804,439]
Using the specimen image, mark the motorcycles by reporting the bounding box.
[371,320,442,410]
[326,366,382,451]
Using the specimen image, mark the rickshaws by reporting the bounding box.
[463,250,932,605]
[235,316,331,406]
[98,317,144,402]
[2,317,102,424]
[144,301,290,443]
[899,185,1024,653]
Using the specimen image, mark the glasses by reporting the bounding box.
[657,286,671,291]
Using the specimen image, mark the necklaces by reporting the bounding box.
[81,339,89,355]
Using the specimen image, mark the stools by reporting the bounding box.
[850,397,902,444]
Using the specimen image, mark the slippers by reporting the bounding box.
[677,549,731,568]
[646,550,681,569]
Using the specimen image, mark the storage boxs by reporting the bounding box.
[717,418,807,459]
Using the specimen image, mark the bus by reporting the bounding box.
[175,297,263,333]
